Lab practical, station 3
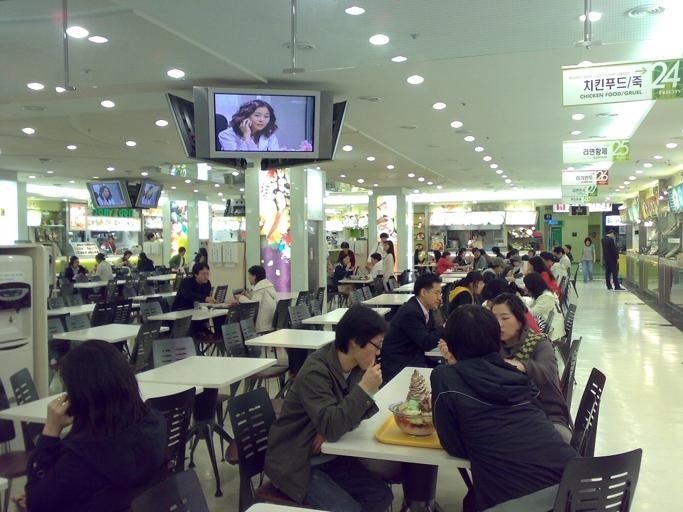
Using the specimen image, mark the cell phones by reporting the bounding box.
[63,394,74,417]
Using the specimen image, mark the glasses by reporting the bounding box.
[368,339,383,351]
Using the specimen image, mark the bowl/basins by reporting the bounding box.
[388,400,439,437]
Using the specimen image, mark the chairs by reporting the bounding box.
[571,367,606,456]
[569,265,579,298]
[227,389,304,510]
[559,335,582,394]
[132,468,208,510]
[485,447,641,509]
[565,302,577,343]
[0,262,570,468]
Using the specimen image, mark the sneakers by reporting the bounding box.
[608,285,626,291]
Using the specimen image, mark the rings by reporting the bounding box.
[57,398,61,400]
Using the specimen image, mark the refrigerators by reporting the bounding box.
[0,253,35,465]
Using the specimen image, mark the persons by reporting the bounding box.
[581,237,596,283]
[232,264,278,338]
[173,264,224,346]
[330,232,574,348]
[66,235,208,300]
[491,293,572,445]
[97,185,115,206]
[141,186,154,205]
[601,230,625,290]
[218,99,279,151]
[24,339,168,512]
[380,274,446,383]
[263,303,395,511]
[430,304,583,511]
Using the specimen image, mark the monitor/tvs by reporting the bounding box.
[86,179,163,209]
[165,93,246,171]
[223,198,245,216]
[194,85,333,160]
[261,100,349,171]
